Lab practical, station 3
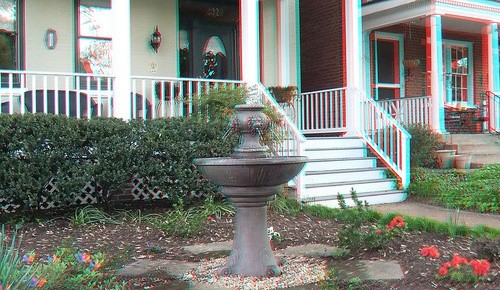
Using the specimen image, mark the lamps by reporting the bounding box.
[152,26,161,53]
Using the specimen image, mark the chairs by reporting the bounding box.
[111,92,152,121]
[19,89,96,120]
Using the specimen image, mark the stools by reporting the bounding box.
[156,97,179,119]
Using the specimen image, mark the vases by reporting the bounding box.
[453,154,471,169]
[437,150,456,169]
